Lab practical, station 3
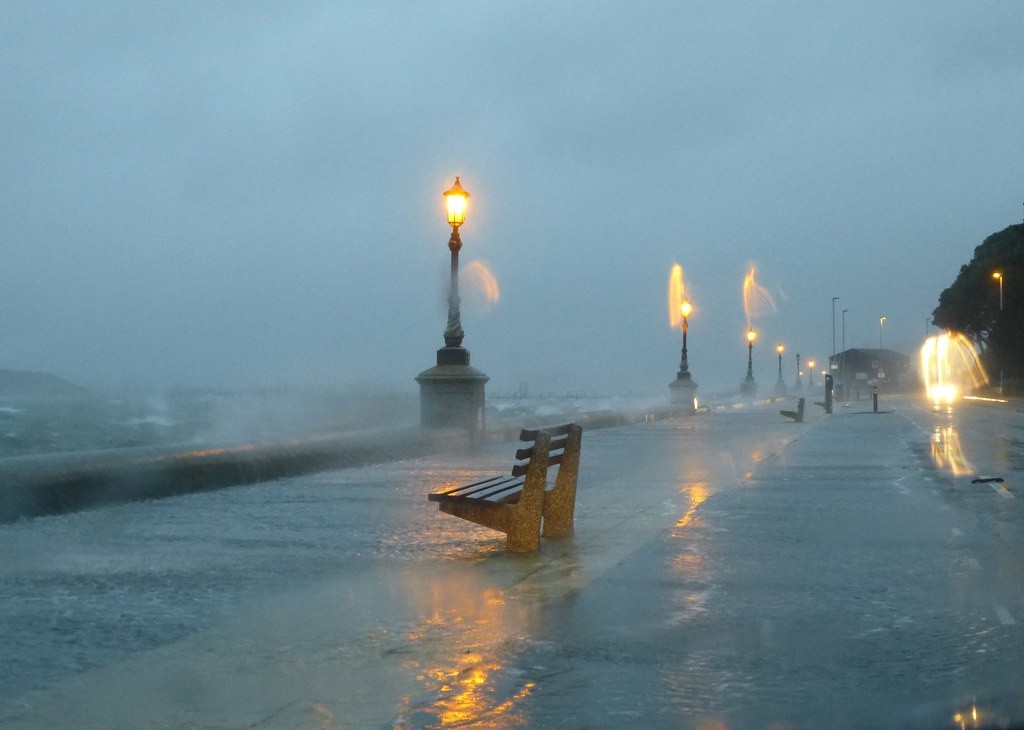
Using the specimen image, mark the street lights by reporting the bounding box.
[667,293,699,411]
[795,353,803,391]
[925,317,933,338]
[841,308,848,367]
[831,297,838,361]
[992,270,1003,396]
[879,315,886,391]
[807,359,817,390]
[414,176,489,429]
[741,327,758,399]
[775,343,787,394]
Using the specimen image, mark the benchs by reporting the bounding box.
[779,398,805,423]
[427,418,584,552]
